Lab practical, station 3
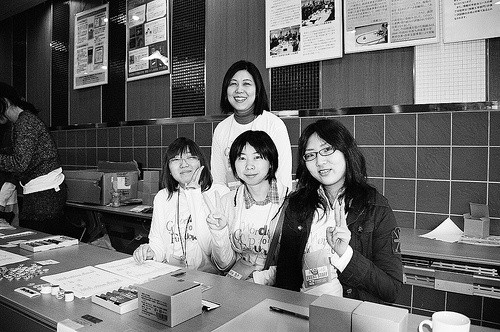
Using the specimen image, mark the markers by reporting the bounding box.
[100,285,138,305]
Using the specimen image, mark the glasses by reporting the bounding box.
[168,157,200,166]
[302,147,338,161]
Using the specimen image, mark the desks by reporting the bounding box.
[398,228,499,300]
[65,202,153,243]
[0,218,500,332]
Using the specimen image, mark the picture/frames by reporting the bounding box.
[124,0,171,82]
[72,5,109,90]
[264,0,343,70]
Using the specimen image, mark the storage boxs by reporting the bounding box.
[138,279,203,328]
[464,203,490,239]
[63,161,159,205]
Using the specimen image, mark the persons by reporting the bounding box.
[271,30,300,51]
[0,85,68,237]
[210,60,293,192]
[302,0,335,21]
[134,137,230,273]
[203,130,290,284]
[274,119,404,302]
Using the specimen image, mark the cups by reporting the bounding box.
[418,310,471,332]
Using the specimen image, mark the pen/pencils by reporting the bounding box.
[269,305,309,321]
